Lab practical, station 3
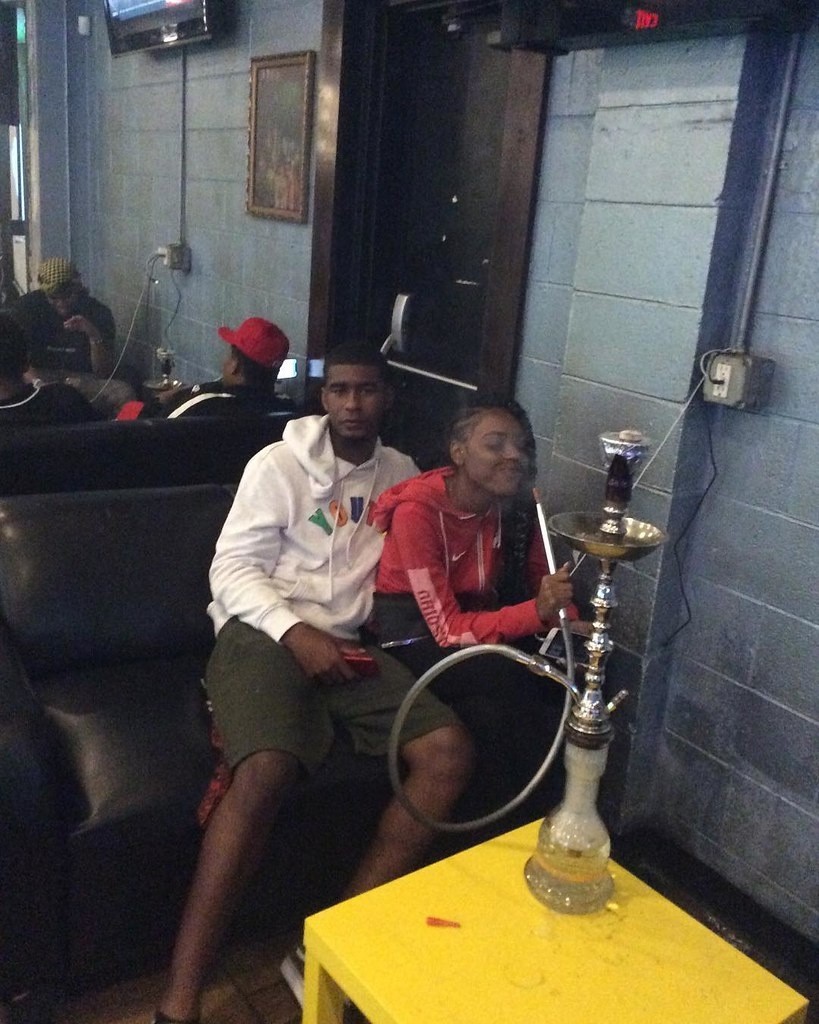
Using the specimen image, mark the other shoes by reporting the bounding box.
[282,946,306,1005]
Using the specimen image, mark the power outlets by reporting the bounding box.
[711,362,733,398]
[157,248,169,264]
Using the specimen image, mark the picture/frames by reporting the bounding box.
[246,48,316,224]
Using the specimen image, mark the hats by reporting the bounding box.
[39,258,79,297]
[219,317,290,371]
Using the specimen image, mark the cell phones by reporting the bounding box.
[342,654,373,664]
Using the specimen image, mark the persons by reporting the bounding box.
[366,399,595,862]
[17,259,133,416]
[155,345,474,1024]
[134,318,290,418]
[0,312,98,424]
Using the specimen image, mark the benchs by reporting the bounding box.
[0,308,563,992]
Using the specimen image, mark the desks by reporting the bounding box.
[300,818,808,1024]
